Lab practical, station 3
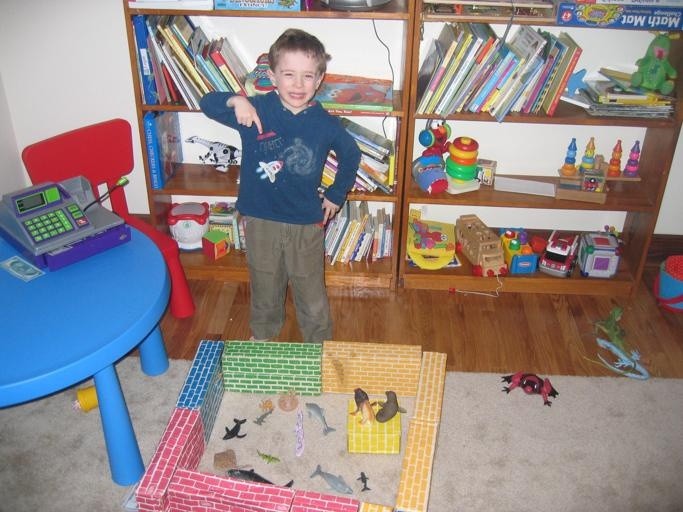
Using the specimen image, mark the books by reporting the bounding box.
[413,21,678,126]
[321,118,394,195]
[233,210,247,250]
[130,13,249,110]
[324,199,392,266]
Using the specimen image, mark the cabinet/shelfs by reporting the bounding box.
[399,0,683,299]
[123,0,416,290]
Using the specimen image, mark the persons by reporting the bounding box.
[200,29,361,344]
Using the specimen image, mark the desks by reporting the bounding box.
[0,226,170,486]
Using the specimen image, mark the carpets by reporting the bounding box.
[0,356,683,512]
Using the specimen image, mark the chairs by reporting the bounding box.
[23,119,196,319]
